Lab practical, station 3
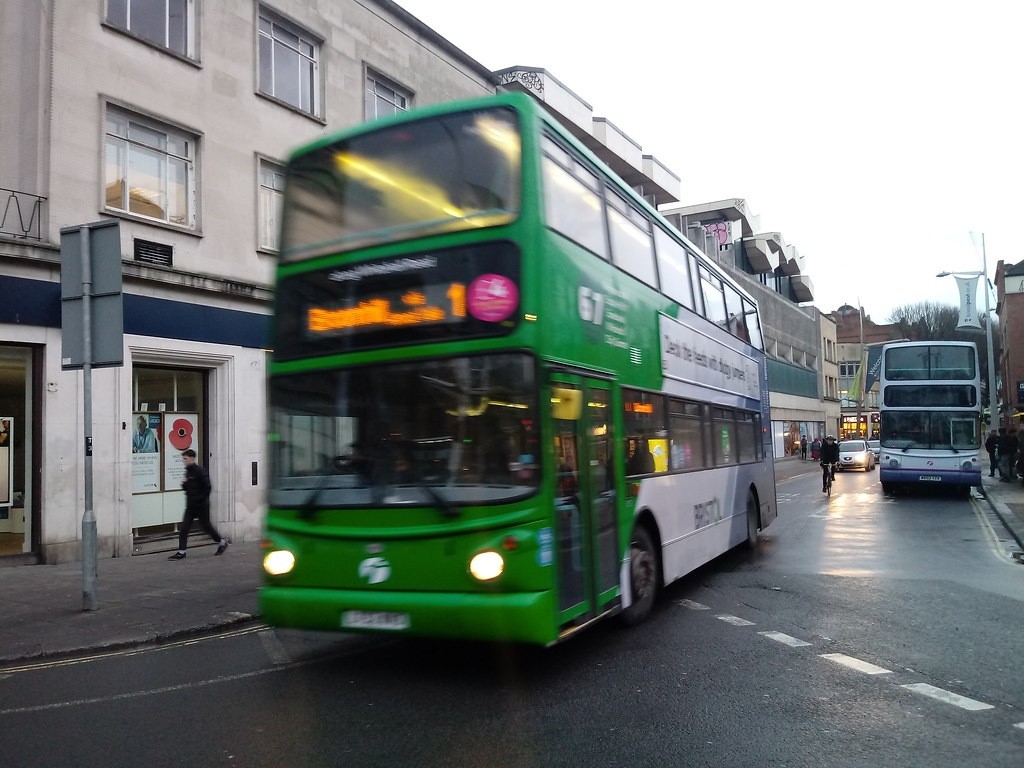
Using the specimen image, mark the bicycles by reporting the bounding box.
[821,462,840,497]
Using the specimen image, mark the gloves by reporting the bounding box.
[820,461,824,466]
[835,461,840,468]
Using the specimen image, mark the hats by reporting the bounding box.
[825,435,836,440]
[803,435,806,437]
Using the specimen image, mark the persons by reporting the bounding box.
[166,450,229,563]
[800,433,823,458]
[984,422,1024,483]
[133,416,157,454]
[347,424,390,485]
[627,440,638,475]
[820,436,839,495]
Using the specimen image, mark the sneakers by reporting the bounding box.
[214,542,228,557]
[168,552,187,561]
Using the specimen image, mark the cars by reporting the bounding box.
[835,440,880,472]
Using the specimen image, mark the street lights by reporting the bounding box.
[936,233,1001,436]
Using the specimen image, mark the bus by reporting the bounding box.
[257,90,777,648]
[879,340,982,497]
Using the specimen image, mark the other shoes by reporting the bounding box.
[830,473,836,481]
[822,485,827,492]
